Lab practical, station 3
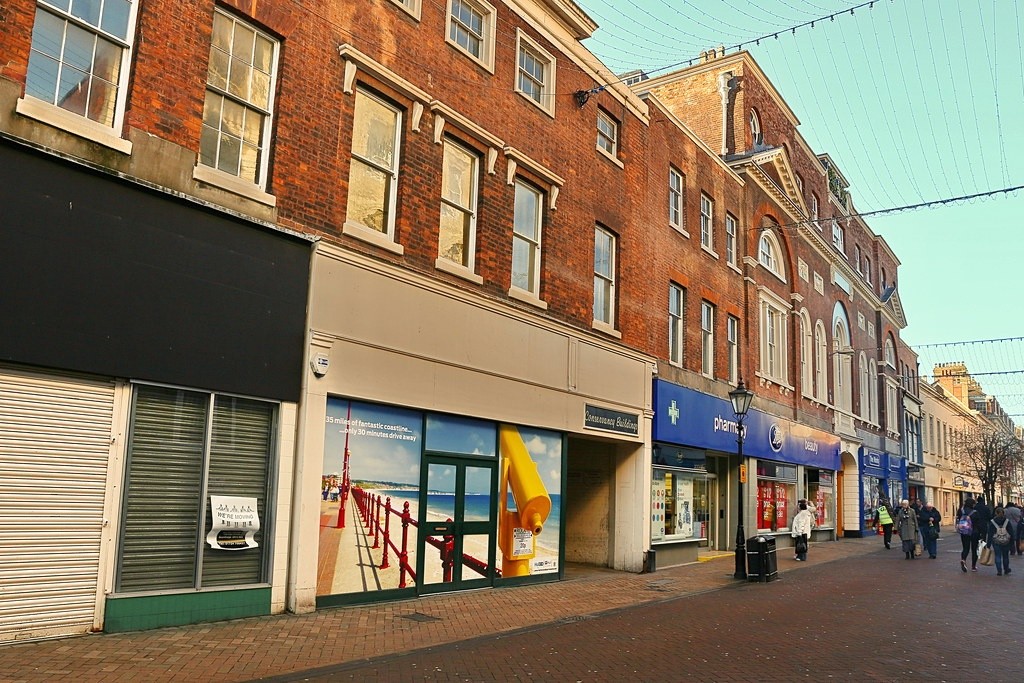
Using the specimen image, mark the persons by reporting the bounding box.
[914,497,929,550]
[956,497,981,573]
[791,498,817,561]
[896,499,919,559]
[974,495,991,549]
[922,500,942,559]
[993,502,1024,556]
[322,481,340,503]
[987,506,1012,575]
[872,500,895,550]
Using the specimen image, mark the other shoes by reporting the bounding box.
[997,572,1002,576]
[1005,569,1011,574]
[887,542,891,549]
[931,556,936,559]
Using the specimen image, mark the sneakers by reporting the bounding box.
[960,560,967,572]
[796,558,801,561]
[793,556,799,559]
[972,567,978,572]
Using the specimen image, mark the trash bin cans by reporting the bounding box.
[747,535,779,581]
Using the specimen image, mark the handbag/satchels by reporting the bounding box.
[985,549,995,566]
[979,545,991,565]
[914,543,922,556]
[976,540,987,559]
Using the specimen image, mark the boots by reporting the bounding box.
[905,552,909,559]
[911,551,915,559]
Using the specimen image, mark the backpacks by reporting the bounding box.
[955,506,977,536]
[990,518,1011,546]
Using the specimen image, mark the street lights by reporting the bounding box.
[728,376,758,578]
